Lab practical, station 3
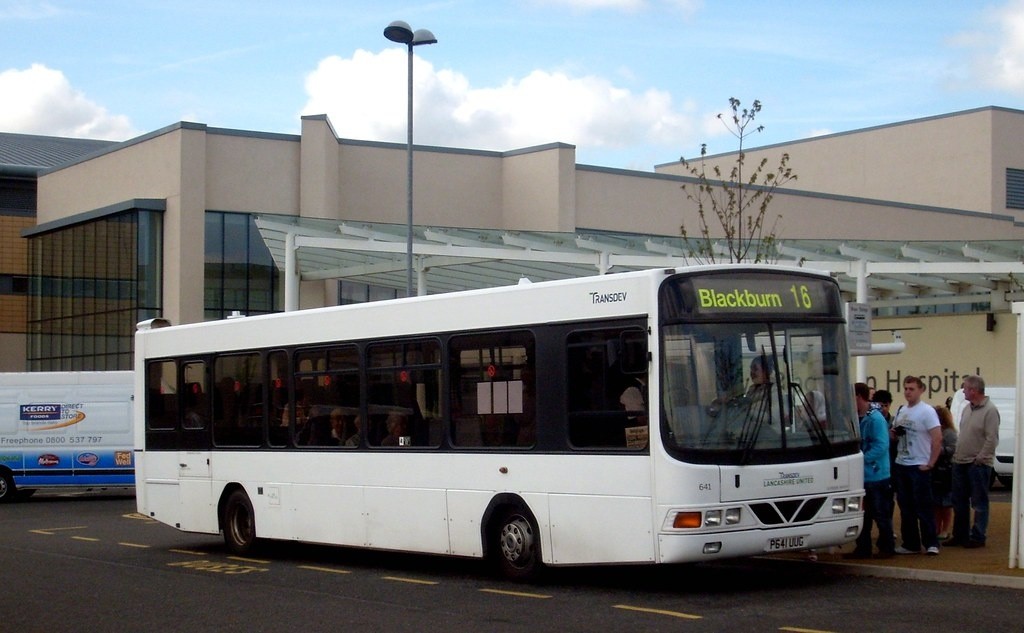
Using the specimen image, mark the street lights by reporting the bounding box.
[383,19,438,293]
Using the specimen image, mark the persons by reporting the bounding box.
[186,365,534,446]
[936,408,957,539]
[889,375,942,555]
[839,382,895,559]
[716,355,779,425]
[618,352,683,435]
[798,391,827,429]
[942,376,1000,548]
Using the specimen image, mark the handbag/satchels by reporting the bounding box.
[918,466,949,496]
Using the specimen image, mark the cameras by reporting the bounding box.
[399,436,411,446]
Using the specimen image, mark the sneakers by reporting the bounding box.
[926,545,941,555]
[894,546,921,554]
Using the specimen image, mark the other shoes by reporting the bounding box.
[874,553,894,559]
[842,549,868,559]
[937,532,953,544]
[941,537,966,547]
[965,540,985,549]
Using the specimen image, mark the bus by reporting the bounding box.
[133,263,865,588]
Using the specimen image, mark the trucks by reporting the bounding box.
[0,371,137,505]
